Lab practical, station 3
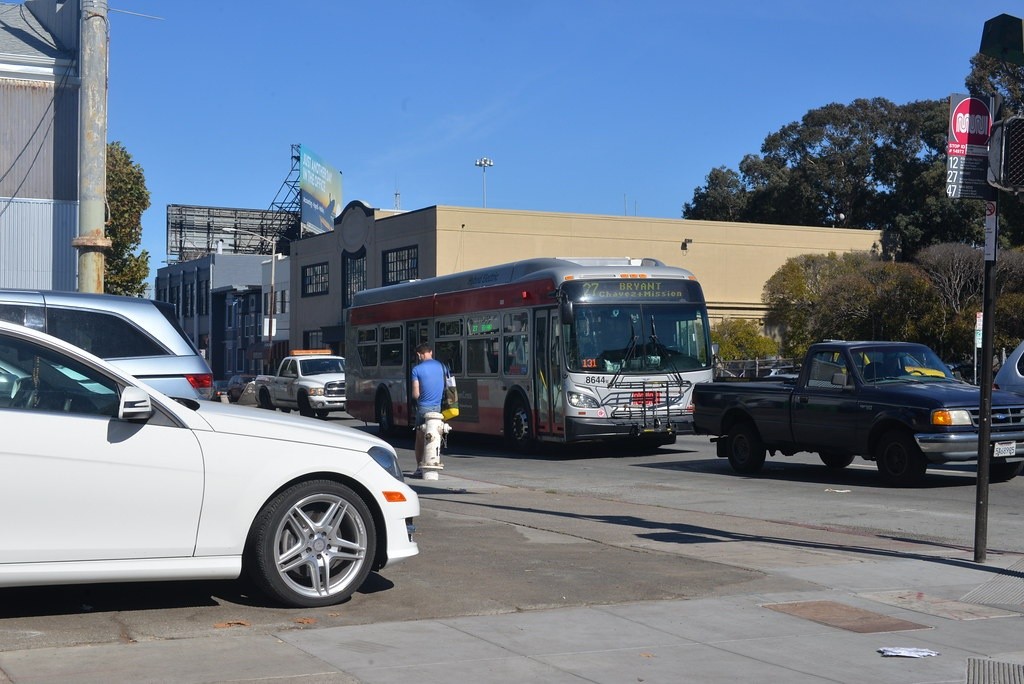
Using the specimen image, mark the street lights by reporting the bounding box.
[475,158,494,208]
[221,227,276,374]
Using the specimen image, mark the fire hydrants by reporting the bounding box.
[412,412,452,481]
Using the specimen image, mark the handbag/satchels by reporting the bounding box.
[441,361,459,421]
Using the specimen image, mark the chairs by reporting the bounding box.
[863,362,894,381]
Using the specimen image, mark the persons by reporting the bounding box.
[875,353,901,378]
[713,357,722,377]
[412,343,450,475]
[639,334,668,360]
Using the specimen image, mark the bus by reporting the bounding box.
[344,256,719,452]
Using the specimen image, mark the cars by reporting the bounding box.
[0,289,217,406]
[0,320,421,607]
[227,374,256,403]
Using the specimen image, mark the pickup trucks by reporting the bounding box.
[254,348,346,417]
[686,338,1023,486]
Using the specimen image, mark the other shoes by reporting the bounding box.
[414,470,422,477]
